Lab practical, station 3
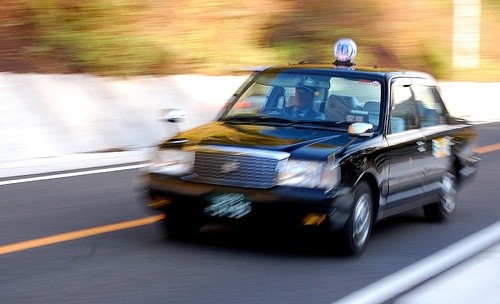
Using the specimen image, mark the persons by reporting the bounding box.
[255,77,325,120]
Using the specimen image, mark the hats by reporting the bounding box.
[296,77,319,95]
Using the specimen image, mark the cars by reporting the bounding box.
[136,39,479,260]
